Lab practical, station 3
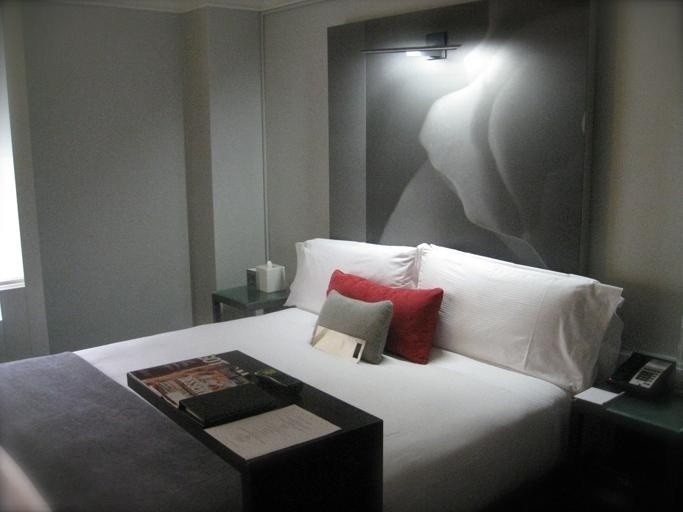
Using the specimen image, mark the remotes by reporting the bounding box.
[253,366,305,394]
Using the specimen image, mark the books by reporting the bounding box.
[178,381,277,429]
[573,386,620,406]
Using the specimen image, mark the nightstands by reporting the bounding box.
[566,373,683,512]
[211,284,291,320]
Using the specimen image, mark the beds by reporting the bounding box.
[0,291,612,512]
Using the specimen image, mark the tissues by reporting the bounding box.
[256,260,286,293]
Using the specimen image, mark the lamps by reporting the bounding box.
[362,31,462,62]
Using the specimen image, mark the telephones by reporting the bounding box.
[609,351,676,398]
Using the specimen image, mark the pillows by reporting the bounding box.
[326,269,442,365]
[283,239,417,315]
[309,289,394,365]
[416,242,605,391]
